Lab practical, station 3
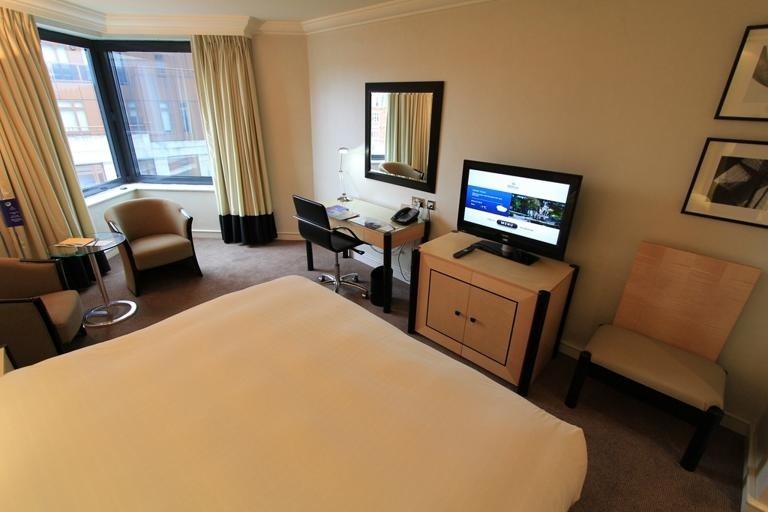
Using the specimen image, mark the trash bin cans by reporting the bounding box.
[370,266,393,306]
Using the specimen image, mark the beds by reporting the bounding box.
[0,272,588,512]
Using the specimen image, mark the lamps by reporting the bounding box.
[337,146,353,202]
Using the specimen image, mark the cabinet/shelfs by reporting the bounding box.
[407,249,551,397]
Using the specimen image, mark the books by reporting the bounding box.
[325,204,360,221]
[60,237,95,248]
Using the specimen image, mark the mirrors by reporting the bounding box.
[364,80,444,194]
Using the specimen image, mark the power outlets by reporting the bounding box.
[412,197,425,209]
[427,200,435,210]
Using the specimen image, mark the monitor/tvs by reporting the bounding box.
[457,159,584,266]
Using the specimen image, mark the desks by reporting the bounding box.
[305,197,431,314]
[45,231,138,328]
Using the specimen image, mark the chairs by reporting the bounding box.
[565,240,763,472]
[0,257,87,370]
[378,162,424,180]
[104,197,204,297]
[292,194,372,299]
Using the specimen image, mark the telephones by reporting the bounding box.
[391,207,420,225]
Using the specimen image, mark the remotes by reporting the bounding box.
[453,245,476,258]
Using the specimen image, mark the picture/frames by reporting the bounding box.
[680,137,768,229]
[714,24,768,123]
